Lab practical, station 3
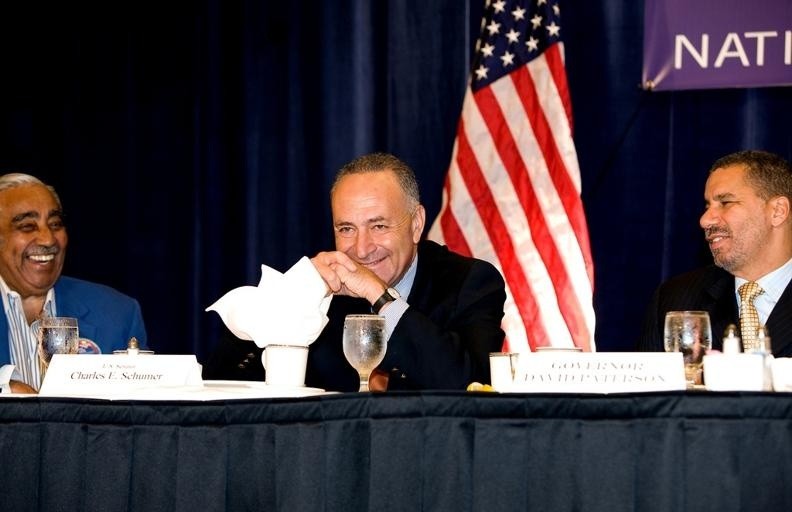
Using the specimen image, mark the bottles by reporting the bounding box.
[126,336,139,356]
[723,324,742,356]
[753,327,774,392]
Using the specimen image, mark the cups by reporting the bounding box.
[261,344,308,387]
[489,351,520,391]
[536,348,582,353]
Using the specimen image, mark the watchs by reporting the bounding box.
[371,288,401,315]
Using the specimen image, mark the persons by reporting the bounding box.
[0,174,147,394]
[229,151,506,390]
[685,149,792,359]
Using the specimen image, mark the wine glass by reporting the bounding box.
[342,313,387,393]
[664,311,712,391]
[38,316,80,368]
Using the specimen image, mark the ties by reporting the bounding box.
[738,278,770,353]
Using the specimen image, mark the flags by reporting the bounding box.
[427,3,599,353]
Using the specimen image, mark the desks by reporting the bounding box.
[0,392,791,512]
